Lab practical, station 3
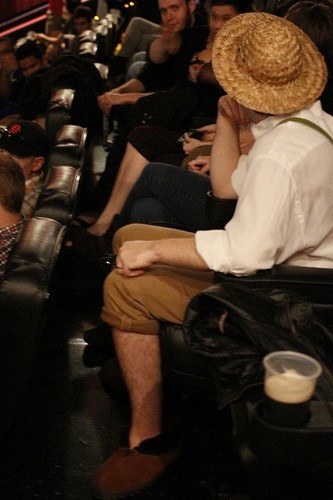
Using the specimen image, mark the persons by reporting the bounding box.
[91,13,333,500]
[0,0,333,280]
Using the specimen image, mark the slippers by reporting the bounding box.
[63,215,108,248]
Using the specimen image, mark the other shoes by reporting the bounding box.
[96,430,179,498]
[83,323,112,344]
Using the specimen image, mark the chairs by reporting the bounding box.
[160,266,333,388]
[1,13,118,331]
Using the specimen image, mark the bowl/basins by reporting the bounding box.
[261,350,322,405]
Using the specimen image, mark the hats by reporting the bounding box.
[212,12,327,114]
[0,120,50,156]
